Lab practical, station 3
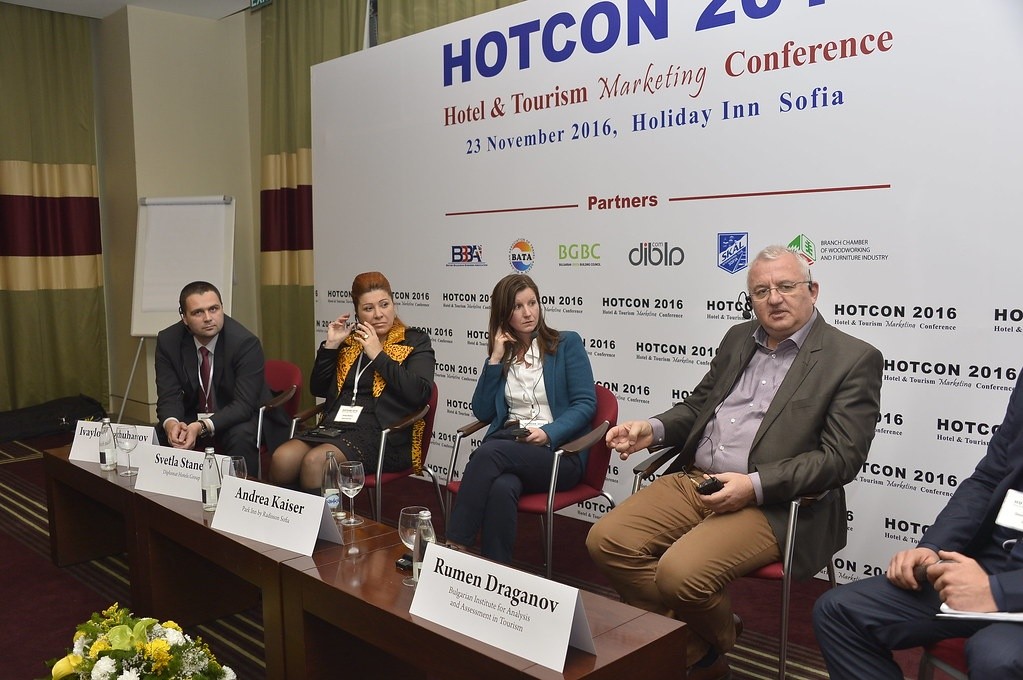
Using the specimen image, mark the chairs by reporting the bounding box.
[633,414,837,679]
[291,374,440,522]
[259,361,307,483]
[447,386,616,582]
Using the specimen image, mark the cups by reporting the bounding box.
[221,455,247,480]
[398,506,429,587]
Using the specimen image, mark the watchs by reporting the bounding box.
[197,420,209,440]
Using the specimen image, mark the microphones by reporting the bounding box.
[181,315,196,337]
[913,559,959,582]
[531,320,542,340]
[742,311,751,319]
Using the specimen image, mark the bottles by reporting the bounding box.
[321,450,343,523]
[200,447,222,512]
[413,510,437,591]
[98,418,118,471]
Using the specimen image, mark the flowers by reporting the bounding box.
[49,600,230,680]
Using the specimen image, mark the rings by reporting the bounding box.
[362,334,368,339]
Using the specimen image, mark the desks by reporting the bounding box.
[44,443,687,680]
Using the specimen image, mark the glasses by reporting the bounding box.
[748,280,813,302]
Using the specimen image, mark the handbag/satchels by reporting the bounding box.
[0,393,107,444]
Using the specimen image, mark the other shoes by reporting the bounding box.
[687,653,731,680]
[732,612,744,639]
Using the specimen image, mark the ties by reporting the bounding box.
[198,347,211,413]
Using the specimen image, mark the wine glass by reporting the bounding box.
[337,461,365,527]
[116,424,138,477]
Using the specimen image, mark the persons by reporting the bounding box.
[813,368,1023,680]
[154,281,292,479]
[269,272,435,496]
[587,247,883,680]
[447,274,597,562]
